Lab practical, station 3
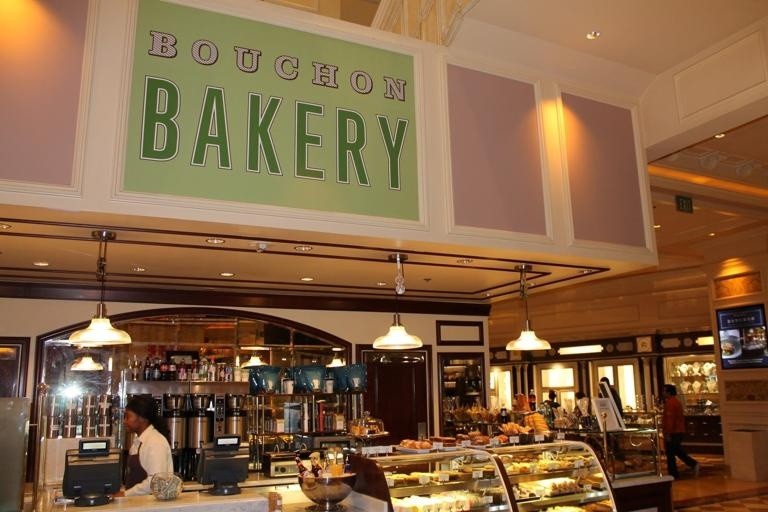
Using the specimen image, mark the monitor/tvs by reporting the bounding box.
[198,446,249,494]
[62,448,122,506]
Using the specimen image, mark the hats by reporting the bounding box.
[126,394,157,419]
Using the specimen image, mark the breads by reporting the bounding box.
[399,414,550,450]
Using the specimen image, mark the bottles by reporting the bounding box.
[529,389,536,410]
[125,354,242,383]
[294,446,352,476]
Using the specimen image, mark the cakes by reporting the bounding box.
[385,464,505,512]
[505,456,614,512]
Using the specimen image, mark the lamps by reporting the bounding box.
[68,230,133,349]
[504,264,552,351]
[370,252,424,352]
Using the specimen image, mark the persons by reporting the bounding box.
[111,394,175,497]
[545,390,560,408]
[662,383,699,480]
[598,376,625,460]
[573,392,591,416]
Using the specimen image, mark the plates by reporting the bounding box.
[437,447,460,452]
[396,446,437,453]
[720,329,741,359]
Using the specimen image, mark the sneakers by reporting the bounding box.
[692,461,700,476]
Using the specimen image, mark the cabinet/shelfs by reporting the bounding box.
[349,446,514,511]
[432,351,723,453]
[565,429,673,512]
[465,440,618,511]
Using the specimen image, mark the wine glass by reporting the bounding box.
[296,473,358,512]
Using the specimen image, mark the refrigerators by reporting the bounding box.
[0,396,32,512]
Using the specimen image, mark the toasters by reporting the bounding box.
[262,451,301,477]
